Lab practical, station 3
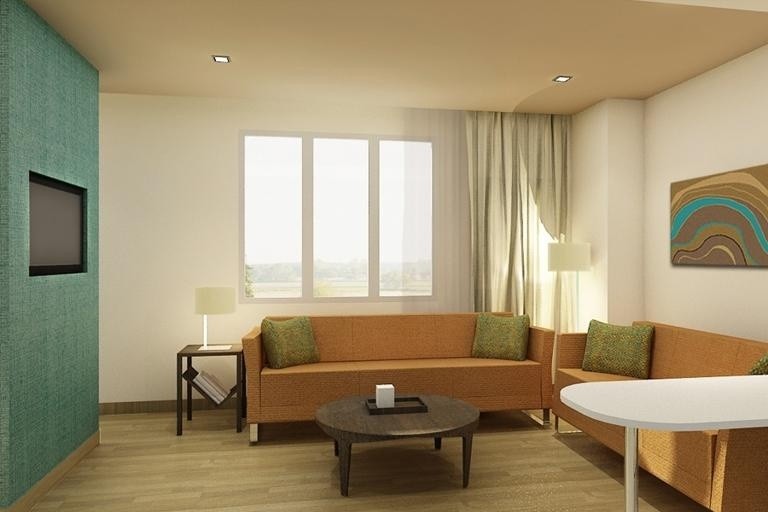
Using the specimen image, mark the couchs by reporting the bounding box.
[552,321,768,512]
[241,311,555,445]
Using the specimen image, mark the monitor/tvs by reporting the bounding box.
[28,170,84,276]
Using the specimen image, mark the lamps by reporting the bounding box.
[195,288,236,350]
[548,241,592,332]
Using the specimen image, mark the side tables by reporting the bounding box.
[177,344,246,435]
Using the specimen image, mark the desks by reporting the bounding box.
[560,375,768,512]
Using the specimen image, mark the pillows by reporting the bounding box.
[262,315,320,368]
[472,313,530,361]
[743,354,768,375]
[581,318,655,379]
